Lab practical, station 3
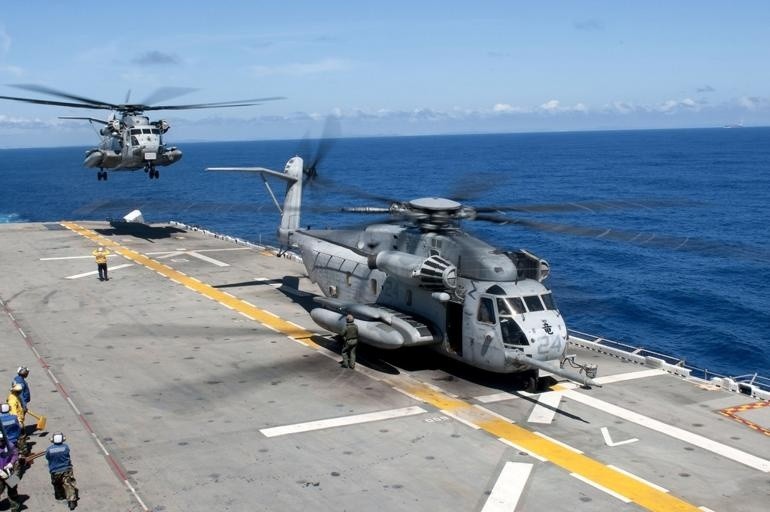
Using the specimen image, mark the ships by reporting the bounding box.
[0,210,770,512]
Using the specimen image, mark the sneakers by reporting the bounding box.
[341,365,354,369]
[55,492,76,510]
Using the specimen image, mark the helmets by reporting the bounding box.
[346,315,354,322]
[12,383,22,391]
[17,366,30,377]
[52,432,63,444]
[1,403,10,413]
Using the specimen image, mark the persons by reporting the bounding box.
[337,314,360,369]
[0,403,22,446]
[91,245,111,282]
[7,365,31,413]
[5,382,25,434]
[0,431,20,504]
[45,431,78,511]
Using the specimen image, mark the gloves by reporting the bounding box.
[4,463,14,473]
[0,470,8,479]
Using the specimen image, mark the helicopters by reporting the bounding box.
[203,115,756,393]
[1,80,285,183]
